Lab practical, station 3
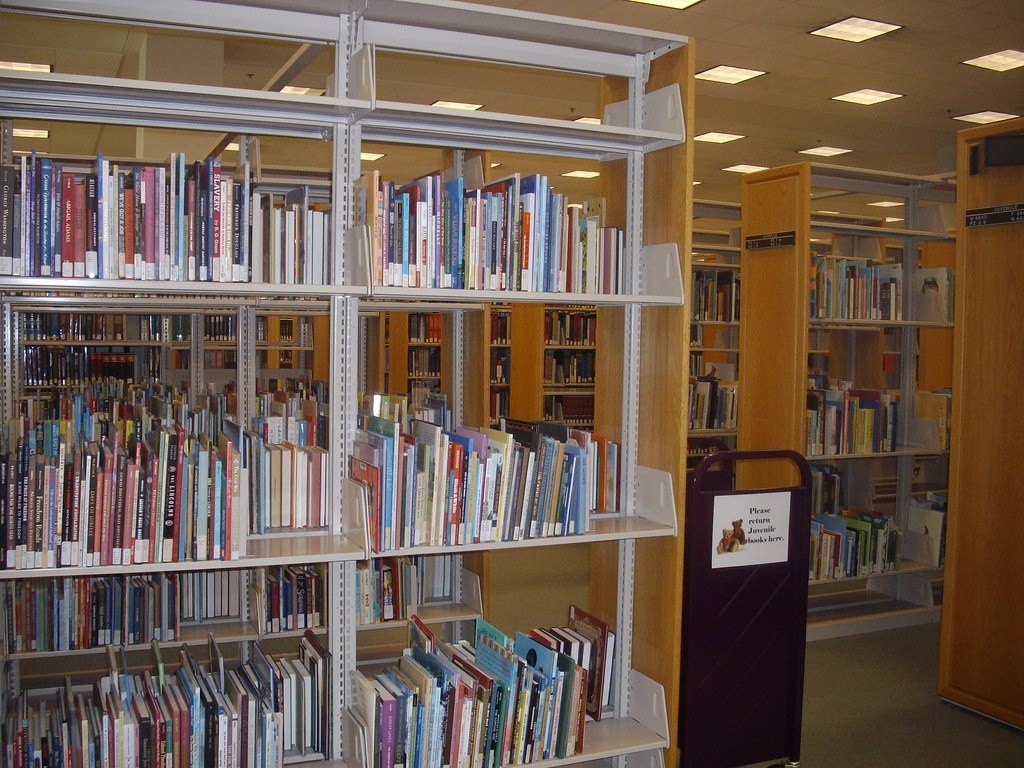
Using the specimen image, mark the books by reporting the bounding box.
[0,554,617,768]
[0,289,622,571]
[362,147,625,296]
[804,373,953,457]
[687,267,741,475]
[808,462,948,584]
[692,253,720,263]
[810,249,955,324]
[0,114,331,287]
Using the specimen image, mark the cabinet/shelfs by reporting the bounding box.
[0,6,691,768]
[733,162,956,646]
[691,199,885,615]
